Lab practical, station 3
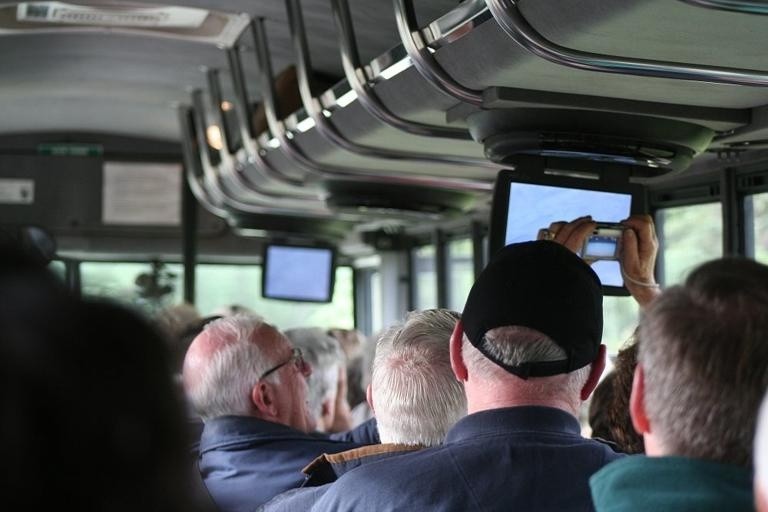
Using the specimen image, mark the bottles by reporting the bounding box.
[460,239,603,381]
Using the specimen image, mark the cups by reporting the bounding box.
[256,346,309,384]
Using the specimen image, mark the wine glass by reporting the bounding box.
[538,226,556,241]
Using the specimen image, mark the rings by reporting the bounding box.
[538,226,556,241]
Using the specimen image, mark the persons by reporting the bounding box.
[0,214,767,511]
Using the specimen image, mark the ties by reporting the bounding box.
[262,242,335,302]
[490,169,648,296]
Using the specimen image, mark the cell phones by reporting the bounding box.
[581,221,625,260]
